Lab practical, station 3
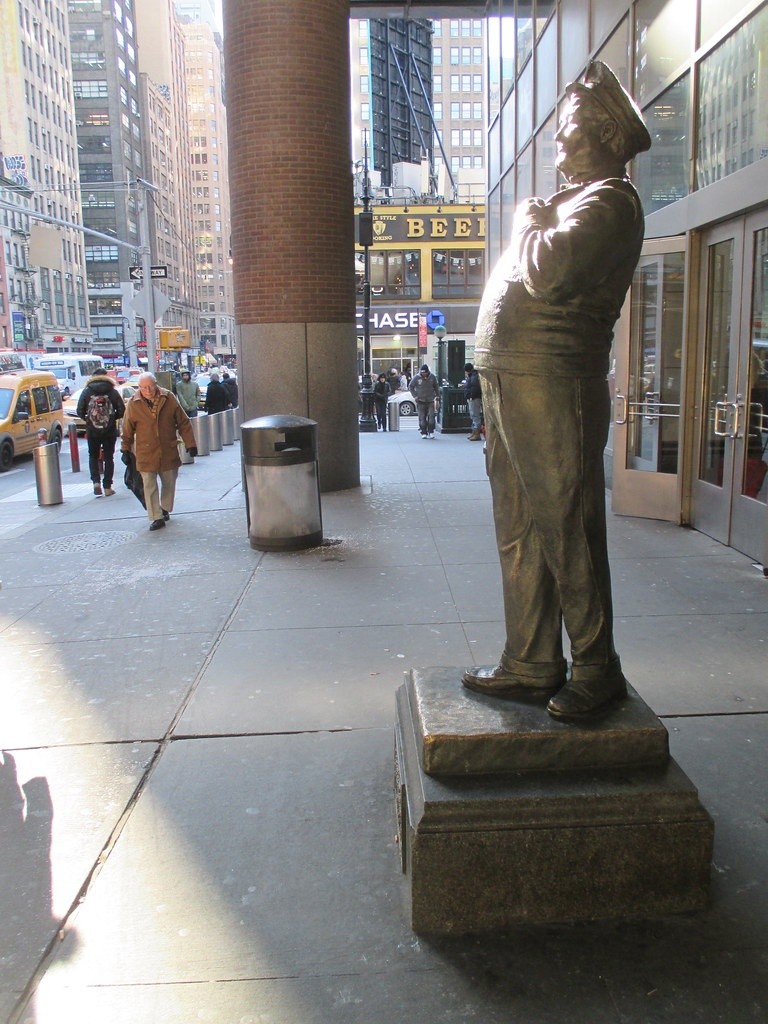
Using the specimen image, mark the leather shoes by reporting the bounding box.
[161,507,170,521]
[149,519,165,530]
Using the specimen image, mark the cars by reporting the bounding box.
[63,367,145,437]
[388,390,482,416]
[195,368,240,410]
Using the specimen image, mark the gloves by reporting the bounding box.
[186,447,198,457]
[121,454,130,465]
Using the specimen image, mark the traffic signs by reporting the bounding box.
[129,266,167,282]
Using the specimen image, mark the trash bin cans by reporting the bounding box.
[387,402,400,432]
[239,414,323,554]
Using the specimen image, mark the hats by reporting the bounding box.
[420,364,428,373]
[463,363,473,371]
[211,373,219,382]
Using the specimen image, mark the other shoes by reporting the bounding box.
[378,423,380,429]
[383,425,386,432]
[93,483,103,495]
[105,486,116,496]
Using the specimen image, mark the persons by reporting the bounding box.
[76,368,126,496]
[387,363,411,391]
[374,373,392,432]
[205,373,239,415]
[410,364,440,439]
[460,363,482,441]
[175,369,201,418]
[120,372,198,531]
[463,62,650,722]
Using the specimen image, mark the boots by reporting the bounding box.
[468,429,481,440]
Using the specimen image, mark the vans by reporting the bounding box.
[0,369,64,472]
[33,353,105,401]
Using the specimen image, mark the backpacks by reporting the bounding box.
[84,385,118,435]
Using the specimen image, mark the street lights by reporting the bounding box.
[121,317,130,366]
[434,325,446,387]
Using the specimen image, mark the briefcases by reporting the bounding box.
[124,452,146,511]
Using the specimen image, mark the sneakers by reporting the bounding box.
[422,434,426,438]
[430,433,435,438]
